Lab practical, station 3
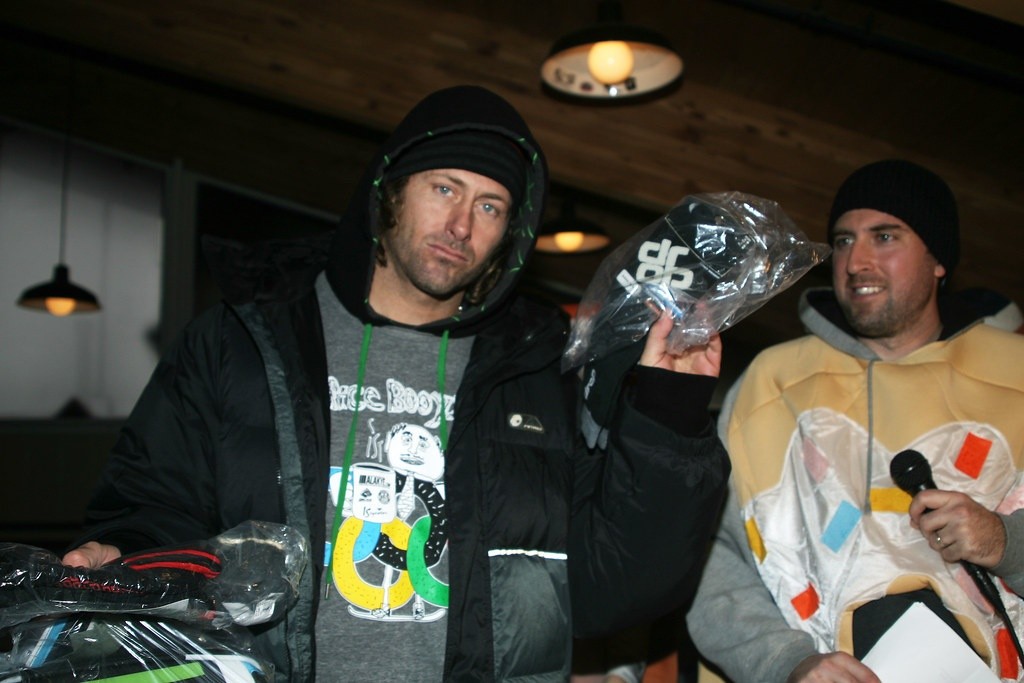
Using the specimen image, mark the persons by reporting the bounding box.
[686,162,1024,683]
[59,83,733,683]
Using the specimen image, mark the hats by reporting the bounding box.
[824,158,963,273]
[384,130,528,203]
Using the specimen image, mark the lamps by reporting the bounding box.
[17,263,101,313]
[539,0,690,107]
[533,202,612,255]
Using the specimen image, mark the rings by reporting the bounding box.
[935,529,945,545]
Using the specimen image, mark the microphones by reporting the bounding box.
[890,450,1005,615]
